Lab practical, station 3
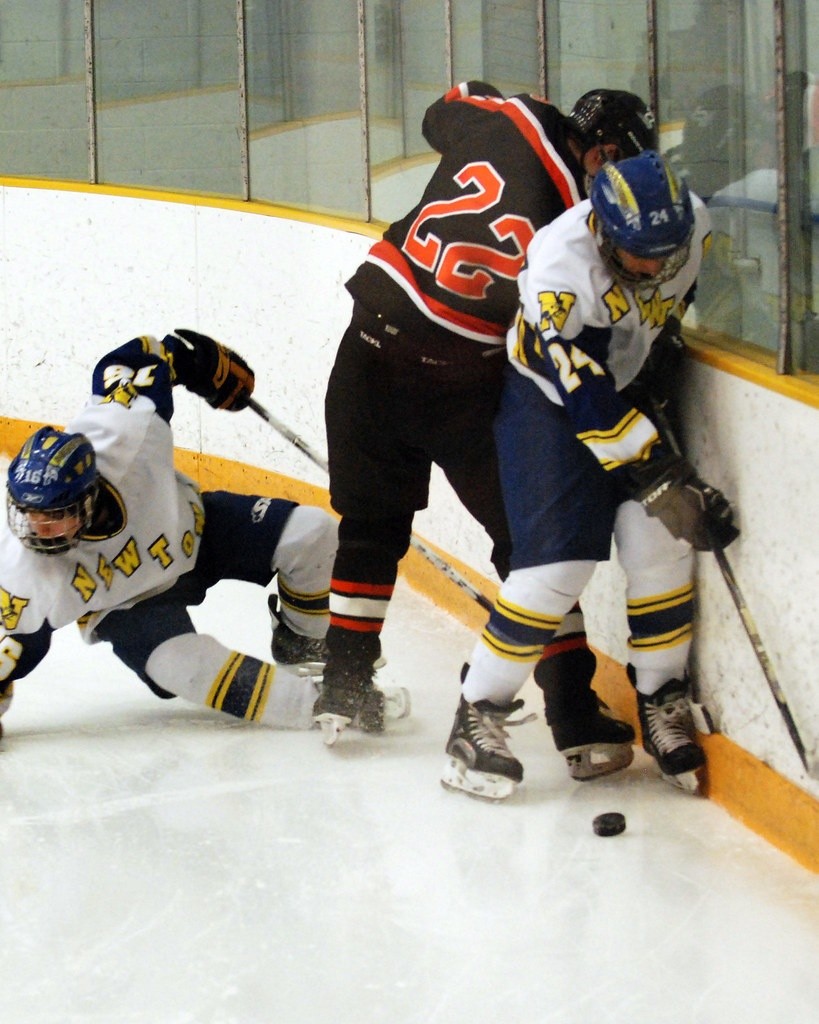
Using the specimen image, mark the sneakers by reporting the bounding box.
[626,663,706,795]
[440,662,538,799]
[268,594,387,677]
[311,642,373,748]
[535,652,636,782]
[314,676,410,732]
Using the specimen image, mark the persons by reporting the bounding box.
[313,80,658,780]
[660,72,819,368]
[0,330,410,743]
[440,154,740,802]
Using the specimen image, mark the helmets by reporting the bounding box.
[587,149,696,289]
[6,426,101,557]
[564,89,658,162]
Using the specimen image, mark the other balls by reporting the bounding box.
[593,813,627,837]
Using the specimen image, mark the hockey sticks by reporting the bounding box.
[687,666,714,735]
[651,397,819,779]
[244,398,494,614]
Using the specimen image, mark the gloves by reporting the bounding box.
[637,316,687,392]
[175,329,255,412]
[631,453,739,551]
